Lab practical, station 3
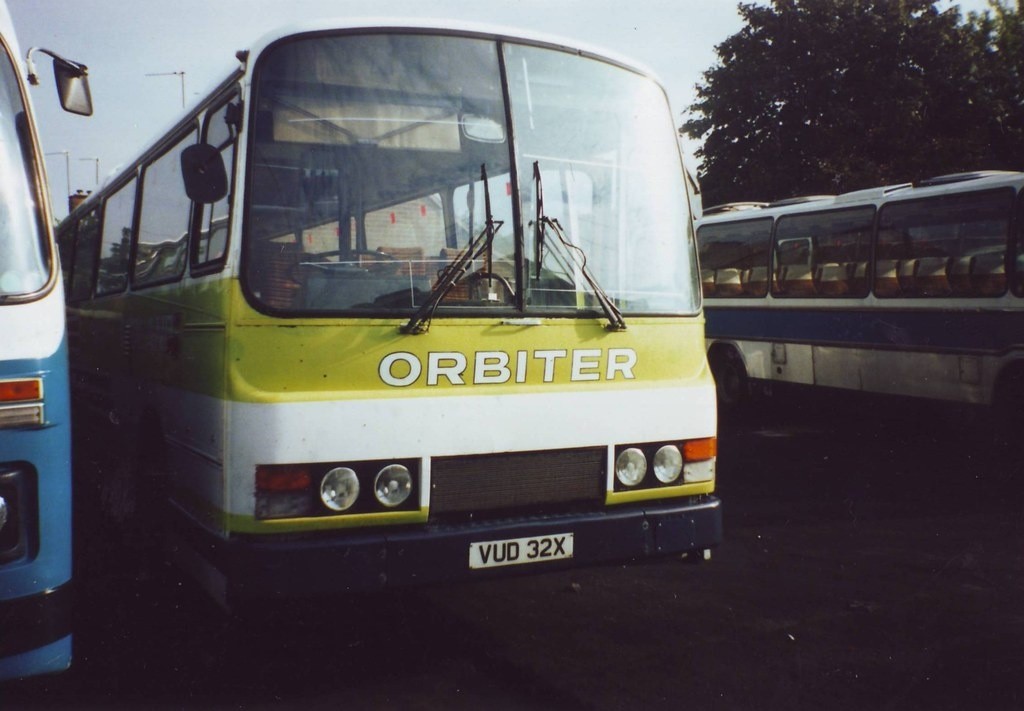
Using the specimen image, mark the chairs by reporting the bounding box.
[700,247,1024,299]
[125,223,485,314]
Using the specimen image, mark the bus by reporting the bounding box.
[693,168,1024,435]
[1,0,95,711]
[56,26,724,574]
